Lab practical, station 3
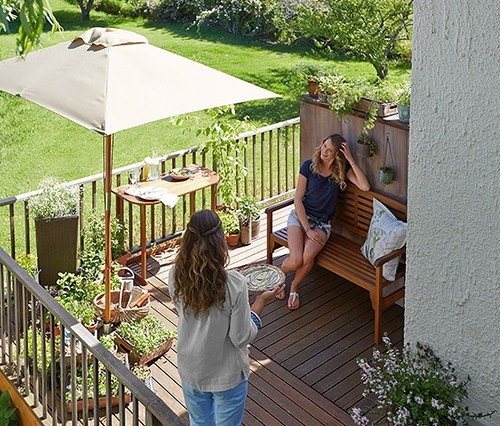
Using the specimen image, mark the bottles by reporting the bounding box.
[143,149,160,180]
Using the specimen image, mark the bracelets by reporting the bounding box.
[351,162,356,166]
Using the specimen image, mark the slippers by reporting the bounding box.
[288,292,300,310]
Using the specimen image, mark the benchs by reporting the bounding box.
[265,185,407,345]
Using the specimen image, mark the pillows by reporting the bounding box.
[359,197,407,282]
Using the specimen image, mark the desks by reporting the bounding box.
[110,163,220,285]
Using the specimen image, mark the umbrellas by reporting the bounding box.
[0,26,283,322]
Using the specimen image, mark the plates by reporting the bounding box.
[139,187,167,200]
[238,264,286,292]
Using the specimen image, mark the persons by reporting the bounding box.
[276,133,371,309]
[168,209,285,426]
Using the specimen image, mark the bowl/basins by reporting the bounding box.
[169,168,193,180]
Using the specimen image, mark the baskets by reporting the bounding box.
[94,281,151,324]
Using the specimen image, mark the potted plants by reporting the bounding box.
[346,81,398,117]
[395,91,410,122]
[316,79,337,103]
[308,68,324,96]
[379,165,393,185]
[27,179,174,413]
[221,193,261,248]
[356,135,374,158]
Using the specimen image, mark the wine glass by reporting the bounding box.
[128,166,141,188]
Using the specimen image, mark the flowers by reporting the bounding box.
[349,332,493,426]
